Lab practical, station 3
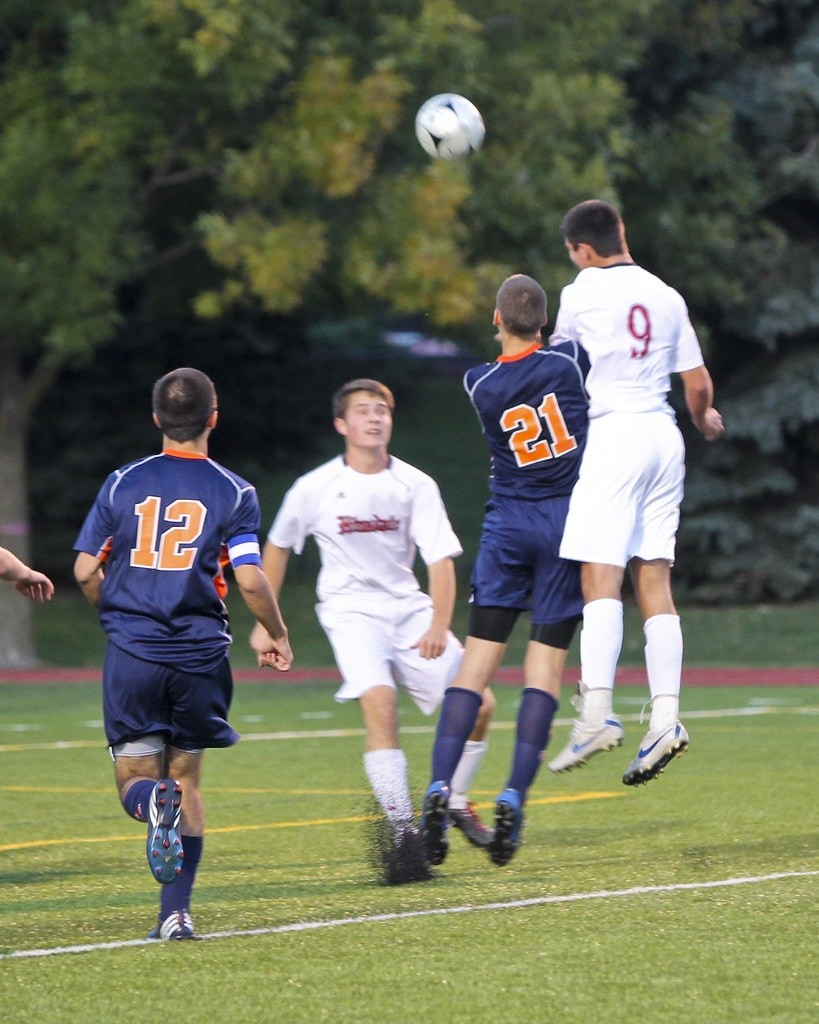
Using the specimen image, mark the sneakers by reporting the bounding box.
[547,713,625,773]
[447,803,495,847]
[623,719,689,786]
[148,907,194,939]
[417,778,452,865]
[489,787,521,867]
[145,777,183,883]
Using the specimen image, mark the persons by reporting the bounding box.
[1,546,57,605]
[418,273,592,865]
[250,376,495,885]
[549,197,728,789]
[71,365,293,941]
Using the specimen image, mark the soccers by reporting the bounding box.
[413,92,487,162]
